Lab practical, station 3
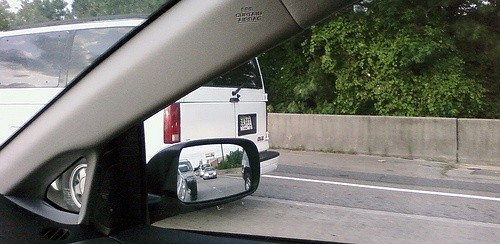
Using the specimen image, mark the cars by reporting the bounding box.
[241,148,251,190]
[176,159,218,203]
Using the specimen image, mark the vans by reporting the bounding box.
[0,13,281,214]
